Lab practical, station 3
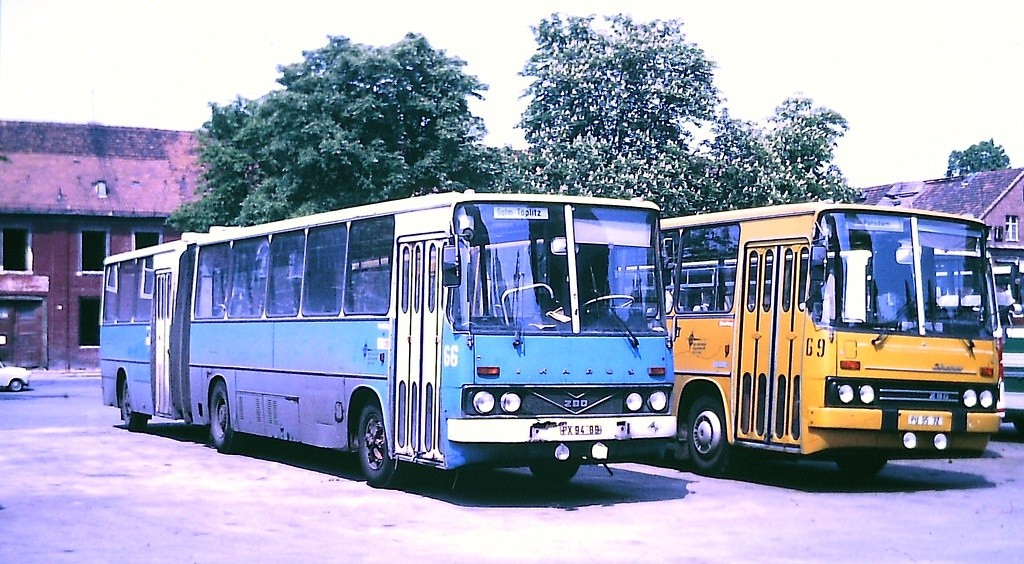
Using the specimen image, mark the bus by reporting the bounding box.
[662,204,1005,477]
[99,189,676,490]
[987,243,1023,433]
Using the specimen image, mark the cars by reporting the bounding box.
[0,361,30,392]
